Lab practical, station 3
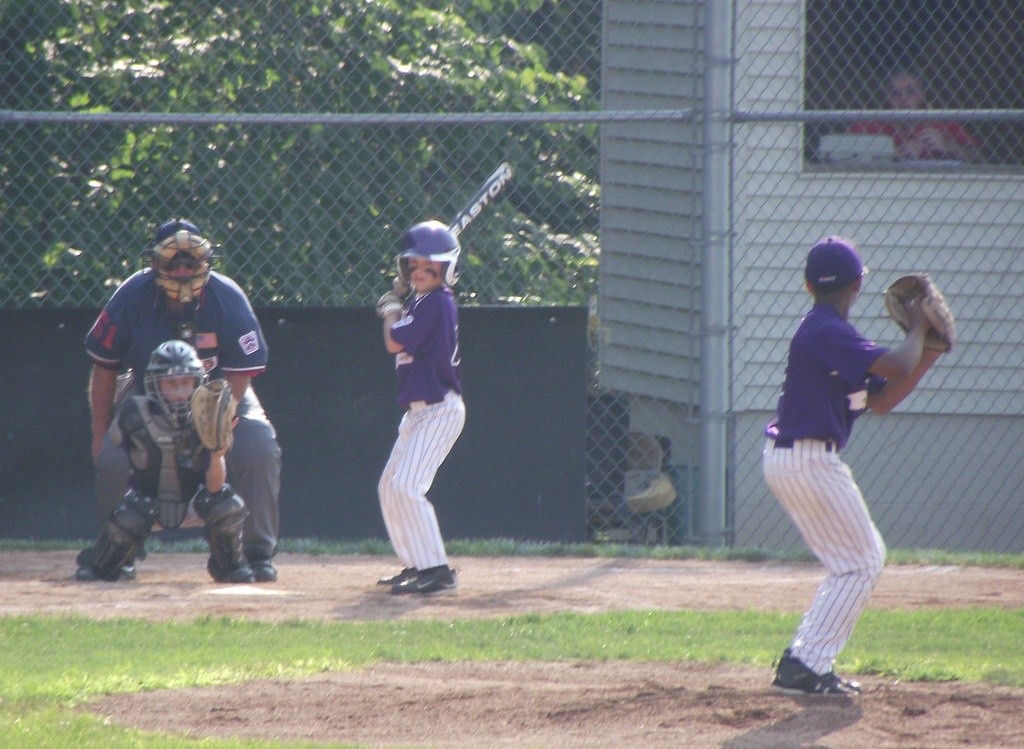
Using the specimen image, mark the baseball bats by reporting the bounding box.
[401,160,516,302]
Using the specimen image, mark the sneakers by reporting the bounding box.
[771,646,863,697]
[377,566,418,588]
[386,564,456,598]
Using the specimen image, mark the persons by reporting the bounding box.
[375,220,467,596]
[72,220,283,584]
[846,71,984,162]
[761,236,954,698]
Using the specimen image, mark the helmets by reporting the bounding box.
[141,217,222,312]
[398,219,461,286]
[143,339,210,429]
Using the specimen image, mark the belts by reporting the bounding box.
[774,437,840,453]
[407,396,443,410]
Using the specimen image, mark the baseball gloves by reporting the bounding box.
[884,270,956,354]
[188,376,238,453]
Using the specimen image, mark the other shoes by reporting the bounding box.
[75,564,97,579]
[121,561,137,578]
[216,566,252,580]
[248,558,278,582]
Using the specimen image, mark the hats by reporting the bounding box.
[805,238,870,288]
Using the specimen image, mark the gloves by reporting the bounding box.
[375,277,409,319]
